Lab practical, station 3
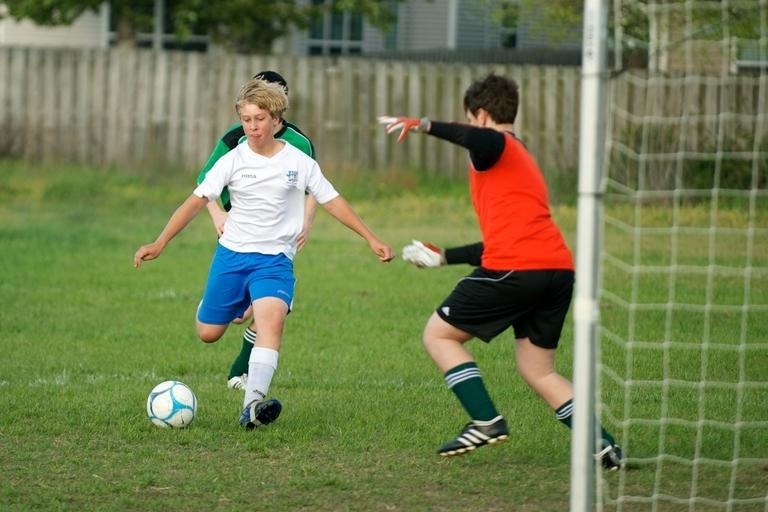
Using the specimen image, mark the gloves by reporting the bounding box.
[377,113,430,145]
[402,239,445,269]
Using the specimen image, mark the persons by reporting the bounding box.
[133,79,394,431]
[377,74,629,472]
[195,70,318,390]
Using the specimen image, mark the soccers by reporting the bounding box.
[145,381,196,430]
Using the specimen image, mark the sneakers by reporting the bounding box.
[592,444,624,473]
[240,399,282,433]
[436,415,511,457]
[225,374,248,391]
